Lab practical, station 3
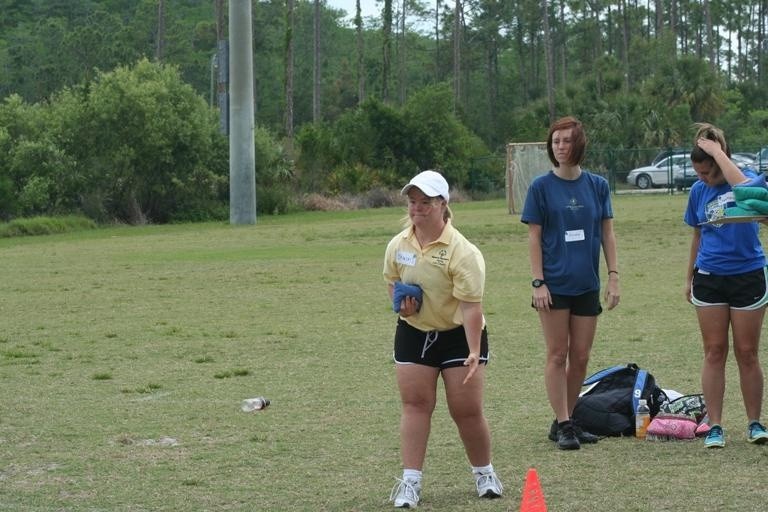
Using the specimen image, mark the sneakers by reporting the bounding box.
[746,422,767,444]
[471,468,503,498]
[389,476,422,510]
[548,418,597,443]
[703,425,725,448]
[556,424,580,450]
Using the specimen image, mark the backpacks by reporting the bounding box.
[572,362,667,438]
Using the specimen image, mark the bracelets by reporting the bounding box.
[607,271,619,275]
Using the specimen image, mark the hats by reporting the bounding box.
[400,170,449,203]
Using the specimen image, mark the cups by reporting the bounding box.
[636,399,651,438]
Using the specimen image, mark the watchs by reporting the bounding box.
[531,279,545,288]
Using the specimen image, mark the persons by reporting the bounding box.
[382,169,504,509]
[684,122,768,448]
[520,117,622,450]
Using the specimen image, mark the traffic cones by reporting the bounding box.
[519,468,548,512]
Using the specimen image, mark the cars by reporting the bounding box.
[627,145,767,191]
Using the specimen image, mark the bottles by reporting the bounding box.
[242,396,270,412]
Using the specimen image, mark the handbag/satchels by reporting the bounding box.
[647,393,708,439]
